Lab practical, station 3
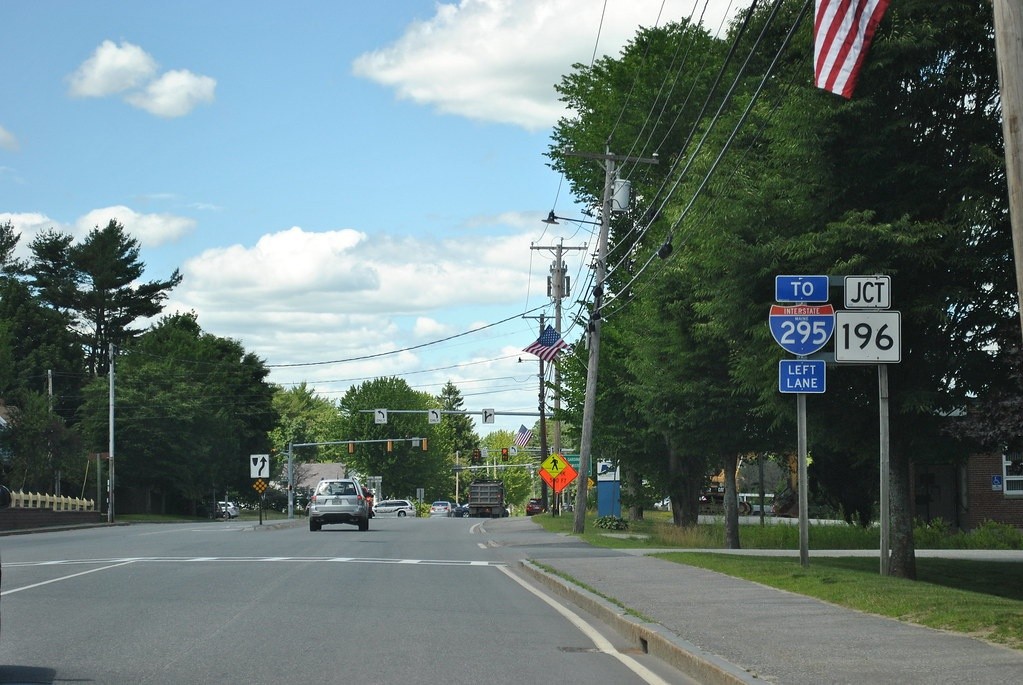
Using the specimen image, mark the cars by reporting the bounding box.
[196,501,240,520]
[429,501,510,518]
[361,486,374,518]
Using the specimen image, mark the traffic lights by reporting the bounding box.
[502,448,508,462]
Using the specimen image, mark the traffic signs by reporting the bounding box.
[428,409,441,424]
[374,409,387,424]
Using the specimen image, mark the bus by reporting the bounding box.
[738,492,794,518]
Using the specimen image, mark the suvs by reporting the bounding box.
[309,477,372,531]
[371,500,416,518]
[526,498,543,516]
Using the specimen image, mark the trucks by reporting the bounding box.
[468,479,506,518]
